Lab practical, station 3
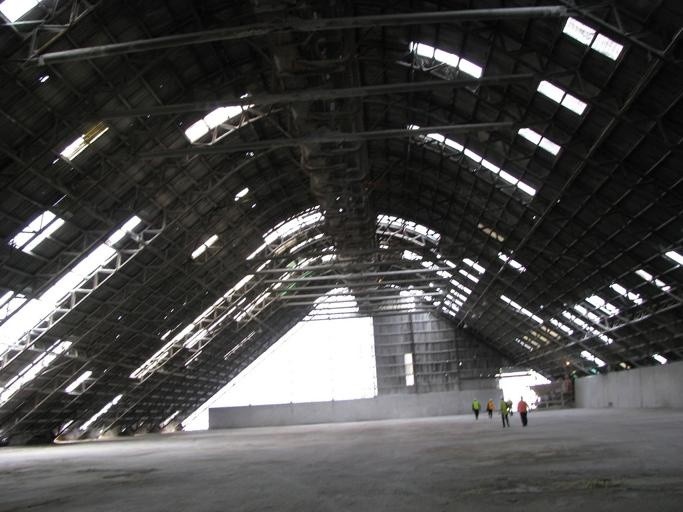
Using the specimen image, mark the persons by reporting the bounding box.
[487,399,496,418]
[507,399,513,416]
[472,398,481,419]
[518,395,529,425]
[500,396,509,426]
